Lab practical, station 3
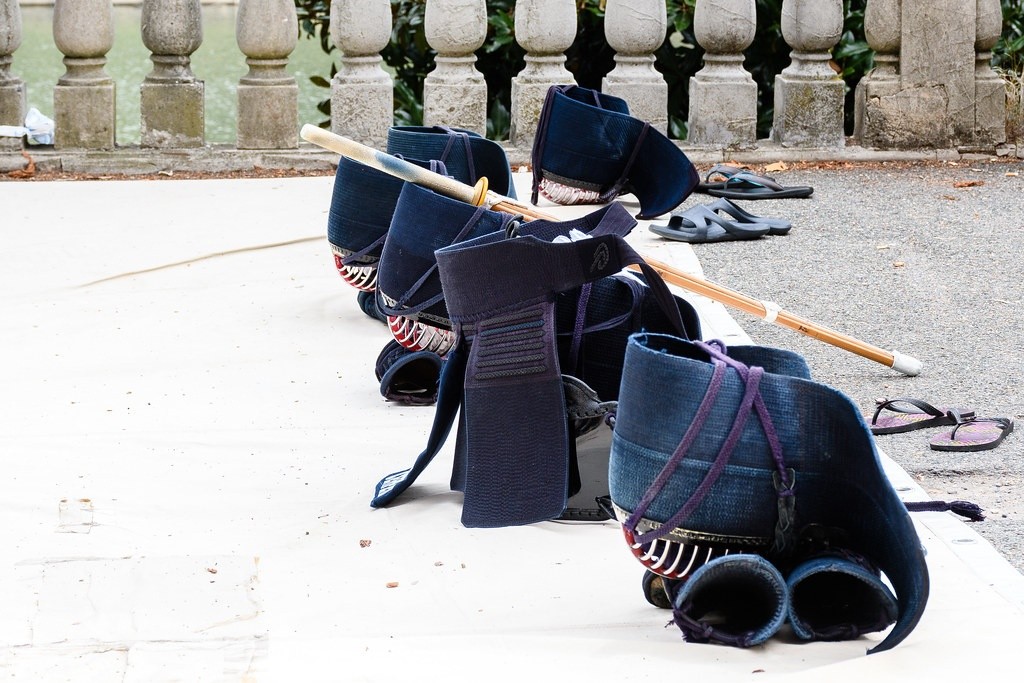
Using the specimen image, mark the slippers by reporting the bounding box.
[867,396,975,435]
[697,163,775,192]
[707,171,814,199]
[682,197,792,234]
[648,202,770,242]
[932,410,1014,451]
